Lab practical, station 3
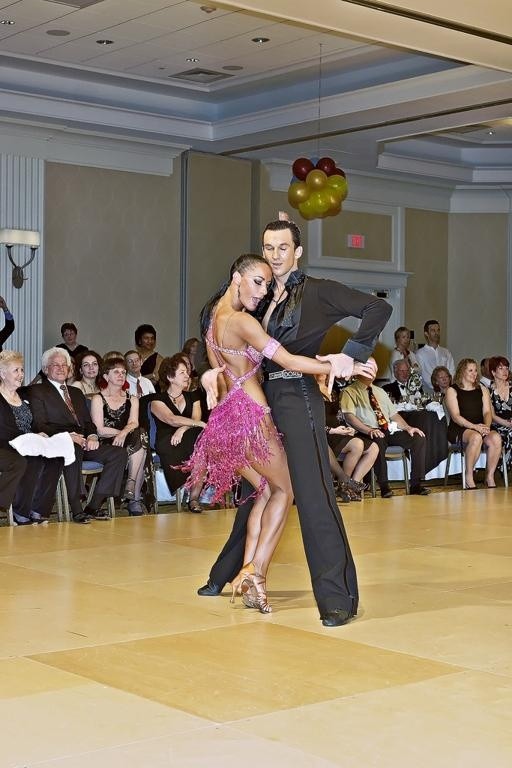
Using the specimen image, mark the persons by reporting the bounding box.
[195,219,392,625]
[0,296,15,353]
[315,314,511,502]
[172,211,376,613]
[1,321,244,526]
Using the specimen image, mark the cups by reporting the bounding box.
[413,391,443,409]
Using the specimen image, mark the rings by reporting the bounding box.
[365,361,368,366]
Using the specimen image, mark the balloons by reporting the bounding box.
[287,155,348,221]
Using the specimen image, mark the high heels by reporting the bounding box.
[126,503,145,515]
[230,562,271,614]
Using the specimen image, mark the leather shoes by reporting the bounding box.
[322,608,357,626]
[188,498,204,513]
[198,579,228,596]
[410,484,431,495]
[72,505,110,524]
[14,513,49,526]
[381,487,393,498]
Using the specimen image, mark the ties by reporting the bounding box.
[60,385,80,427]
[137,379,143,395]
[367,386,388,431]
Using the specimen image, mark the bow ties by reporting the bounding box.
[401,384,405,389]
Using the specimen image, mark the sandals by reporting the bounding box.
[335,477,370,501]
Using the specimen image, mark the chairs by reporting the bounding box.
[336,454,364,499]
[148,402,181,514]
[61,461,116,522]
[371,446,410,498]
[8,478,62,526]
[442,396,509,490]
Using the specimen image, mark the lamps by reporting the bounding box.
[0,228,40,288]
